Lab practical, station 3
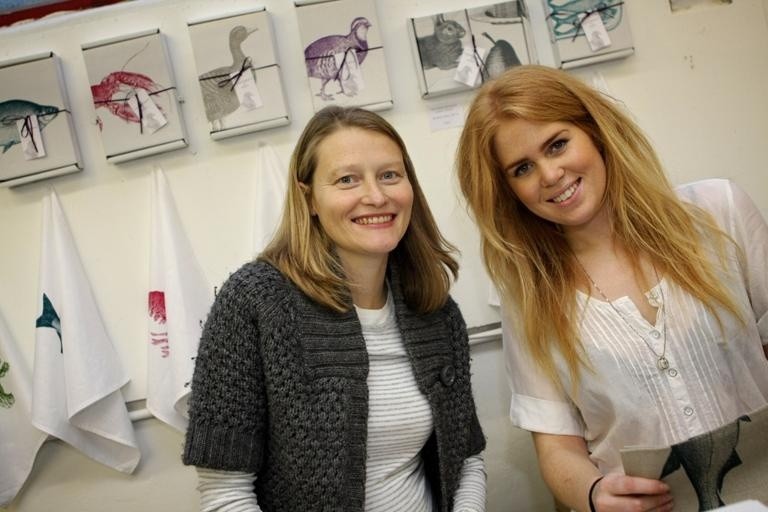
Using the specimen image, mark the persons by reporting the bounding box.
[179,104,489,512]
[457,65,767,512]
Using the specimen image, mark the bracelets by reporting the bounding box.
[589,475,603,511]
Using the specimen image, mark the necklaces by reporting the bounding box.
[563,231,669,369]
